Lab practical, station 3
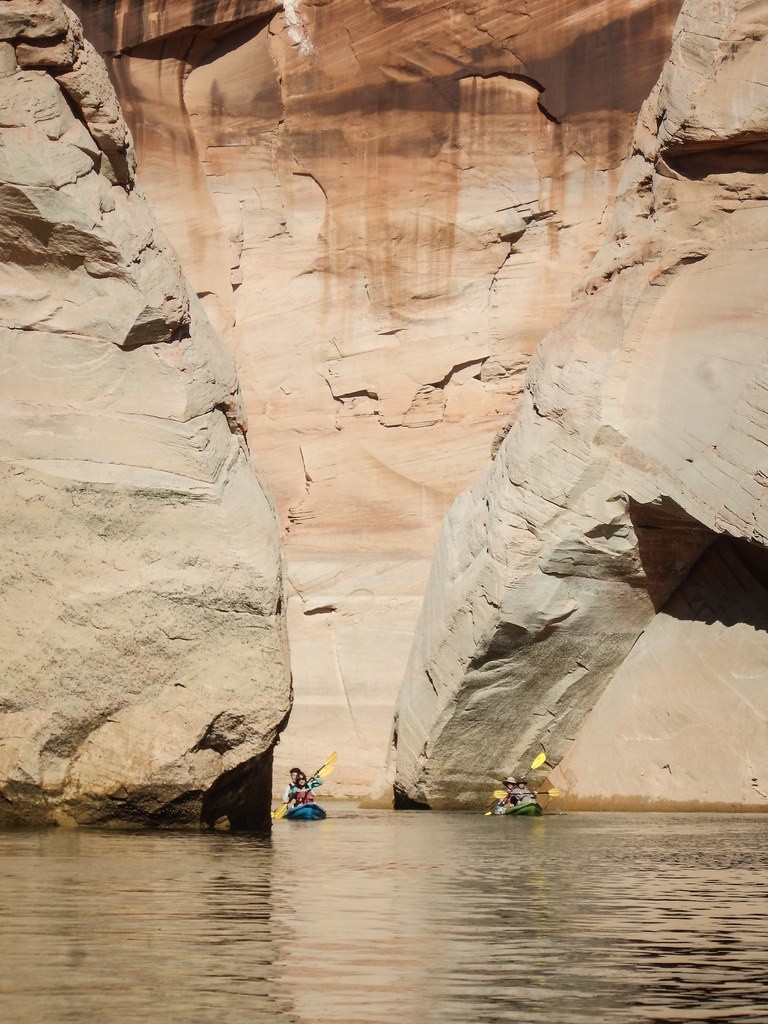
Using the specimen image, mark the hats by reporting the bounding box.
[503,777,516,786]
[516,779,528,785]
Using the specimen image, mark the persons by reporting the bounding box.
[510,778,538,808]
[282,768,302,804]
[501,776,516,808]
[288,772,323,809]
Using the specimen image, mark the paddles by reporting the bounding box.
[493,788,561,800]
[272,750,339,820]
[484,753,546,816]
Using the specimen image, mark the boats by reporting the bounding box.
[494,800,543,816]
[282,801,327,820]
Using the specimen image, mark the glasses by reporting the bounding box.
[299,778,304,780]
